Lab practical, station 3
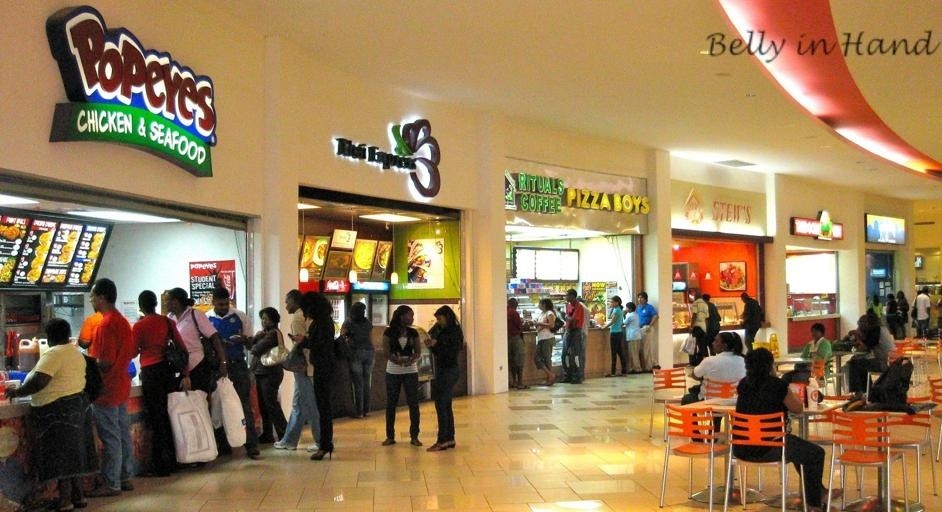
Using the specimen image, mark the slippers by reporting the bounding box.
[512,385,517,388]
[518,385,531,389]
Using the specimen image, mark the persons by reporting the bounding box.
[572,295,593,380]
[681,331,746,444]
[381,305,422,446]
[507,297,533,389]
[556,288,585,383]
[341,301,375,420]
[531,296,558,386]
[6,277,334,510]
[635,291,659,373]
[847,307,896,402]
[688,288,711,366]
[738,292,762,353]
[622,302,643,373]
[869,285,942,341]
[780,322,835,383]
[842,314,870,394]
[701,293,721,357]
[600,295,627,378]
[732,346,846,511]
[424,304,464,452]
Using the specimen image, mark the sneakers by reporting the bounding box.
[306,442,319,452]
[59,503,74,511]
[274,438,297,449]
[73,498,88,508]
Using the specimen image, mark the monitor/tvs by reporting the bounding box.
[915,256,922,268]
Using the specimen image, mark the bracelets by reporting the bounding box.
[845,332,853,338]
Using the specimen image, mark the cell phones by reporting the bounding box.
[288,333,295,340]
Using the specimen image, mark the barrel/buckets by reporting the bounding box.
[39,339,52,356]
[19,338,39,374]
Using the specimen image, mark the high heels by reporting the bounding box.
[311,445,333,460]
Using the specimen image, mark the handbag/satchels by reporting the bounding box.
[83,352,105,402]
[868,356,913,404]
[166,317,188,371]
[167,389,219,464]
[216,376,247,448]
[911,296,917,318]
[191,308,221,370]
[260,329,289,367]
[680,333,696,356]
[546,309,564,333]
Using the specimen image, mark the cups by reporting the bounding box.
[806,384,819,411]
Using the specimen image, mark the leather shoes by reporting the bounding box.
[383,438,395,444]
[122,480,134,490]
[448,440,455,447]
[411,438,422,446]
[84,484,121,497]
[248,447,259,456]
[427,441,448,451]
